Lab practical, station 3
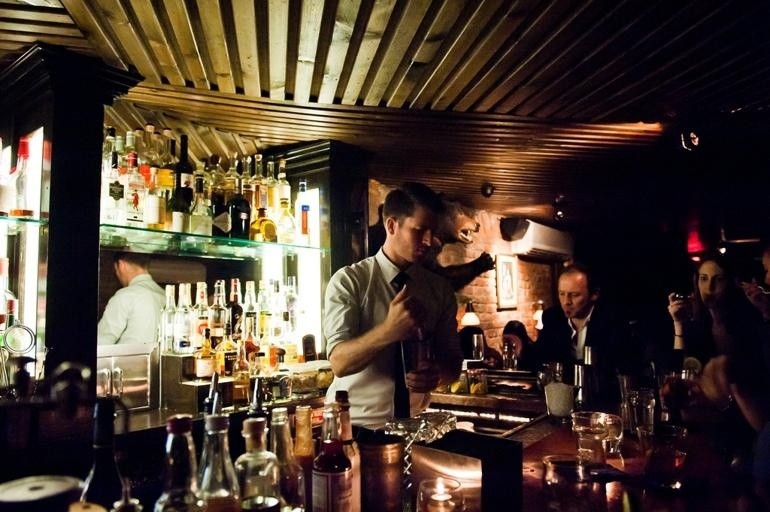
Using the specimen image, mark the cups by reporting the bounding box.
[411,476,467,512]
[669,287,697,322]
[501,338,520,370]
[471,334,485,358]
[539,354,710,510]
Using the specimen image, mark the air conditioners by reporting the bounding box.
[512,218,576,262]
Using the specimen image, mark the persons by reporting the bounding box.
[501,318,537,368]
[664,345,769,512]
[96,248,175,345]
[741,251,769,318]
[323,184,465,434]
[664,258,770,371]
[542,266,640,414]
[455,326,502,370]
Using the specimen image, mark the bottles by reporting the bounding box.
[72,384,366,512]
[0,256,19,331]
[159,276,307,404]
[1,136,36,216]
[97,117,313,246]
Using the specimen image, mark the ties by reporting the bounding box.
[393,270,412,420]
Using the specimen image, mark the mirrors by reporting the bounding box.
[98,249,251,416]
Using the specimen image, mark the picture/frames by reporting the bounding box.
[495,254,519,311]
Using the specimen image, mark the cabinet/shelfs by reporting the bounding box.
[98,354,150,414]
[161,352,234,414]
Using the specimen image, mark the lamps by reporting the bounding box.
[460,297,481,326]
